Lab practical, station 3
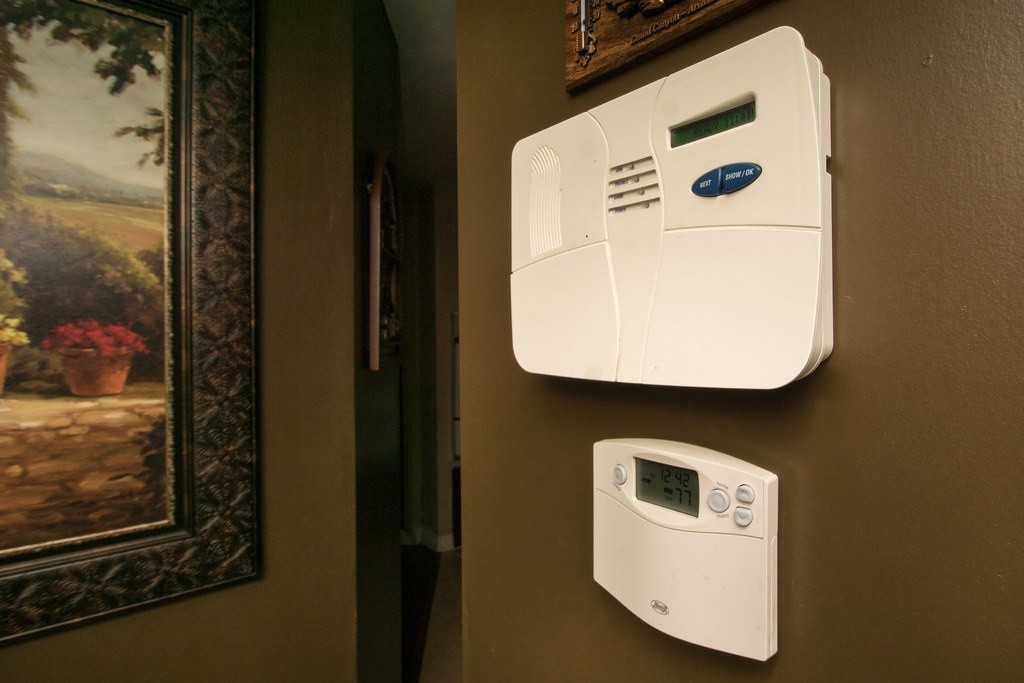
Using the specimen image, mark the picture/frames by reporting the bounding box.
[0,0,267,647]
[563,0,751,96]
